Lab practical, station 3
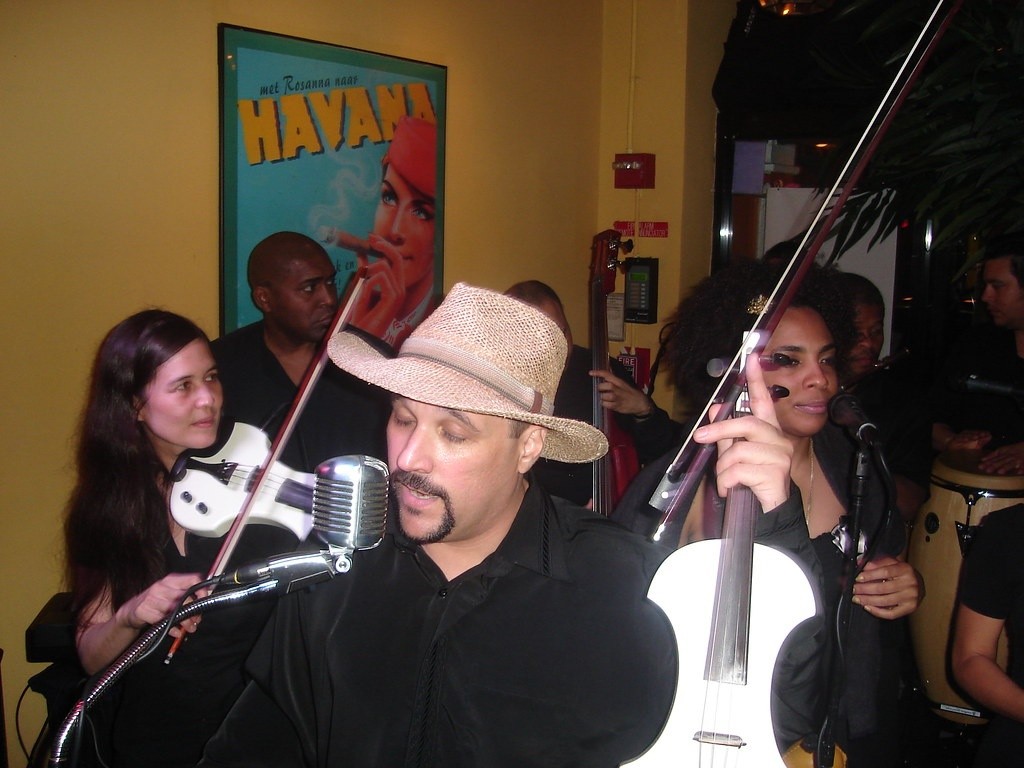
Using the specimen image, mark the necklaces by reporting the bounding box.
[805,438,813,525]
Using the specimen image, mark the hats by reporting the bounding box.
[327,282,609,463]
[382,117,439,200]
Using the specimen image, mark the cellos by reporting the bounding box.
[584,228,641,518]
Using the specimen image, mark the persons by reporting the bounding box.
[932,231,1024,474]
[65,310,280,768]
[203,281,825,768]
[210,231,398,474]
[503,279,672,509]
[605,260,926,768]
[834,273,933,520]
[952,503,1024,768]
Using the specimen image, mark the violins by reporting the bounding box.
[165,417,318,544]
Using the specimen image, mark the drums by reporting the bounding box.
[905,454,1024,729]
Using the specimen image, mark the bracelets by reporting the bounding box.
[634,396,654,419]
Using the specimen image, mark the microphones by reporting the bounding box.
[828,393,881,451]
[214,455,390,593]
[945,368,1024,397]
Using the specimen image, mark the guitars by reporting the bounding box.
[617,328,825,768]
